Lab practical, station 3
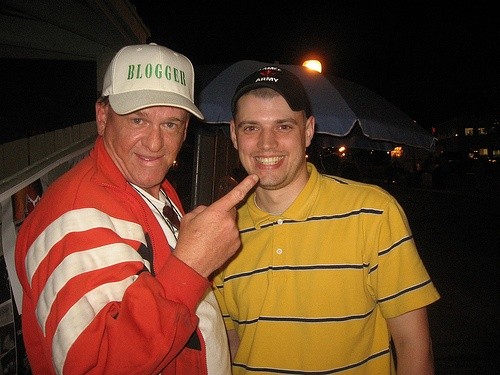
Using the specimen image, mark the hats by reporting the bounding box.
[230,66,311,119]
[101,41,204,121]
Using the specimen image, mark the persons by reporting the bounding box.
[15,43,259,375]
[208,66,441,375]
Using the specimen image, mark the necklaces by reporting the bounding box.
[128,181,182,242]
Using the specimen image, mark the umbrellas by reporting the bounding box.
[194,60,438,151]
